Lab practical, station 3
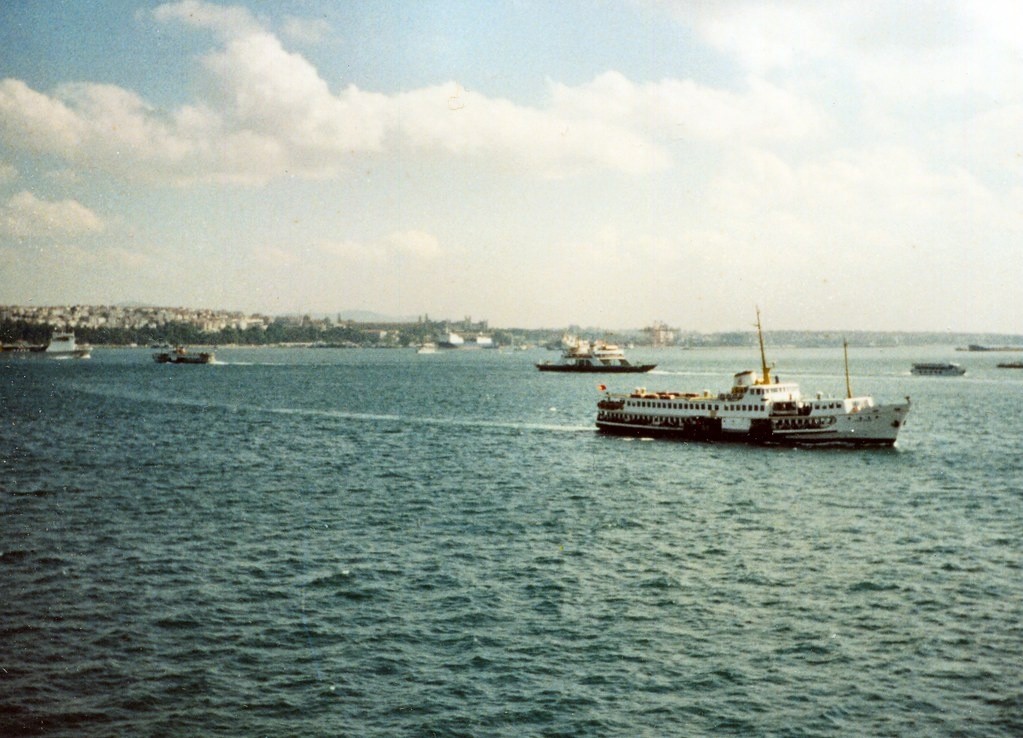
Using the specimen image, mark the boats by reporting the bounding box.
[0,328,93,363]
[998,362,1023,368]
[955,344,1022,352]
[151,345,217,365]
[534,337,656,373]
[413,329,532,355]
[593,307,914,454]
[910,361,965,377]
